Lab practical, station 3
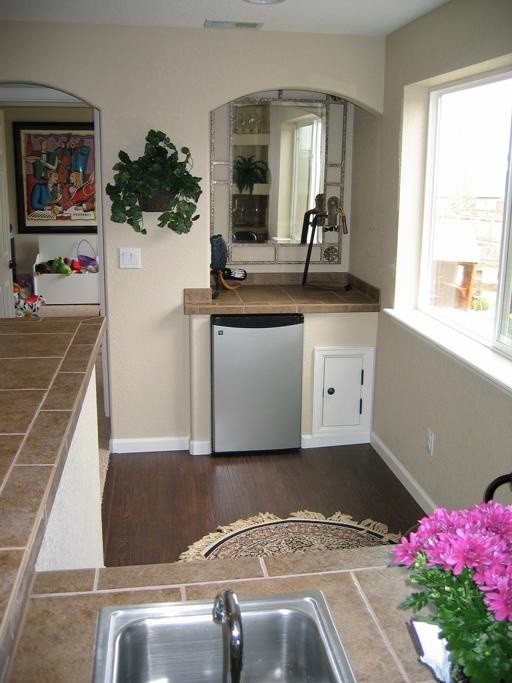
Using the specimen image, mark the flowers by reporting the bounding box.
[386,501,512,682]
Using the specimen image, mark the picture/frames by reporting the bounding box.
[12,119,97,235]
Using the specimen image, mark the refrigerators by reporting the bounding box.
[210,311,305,453]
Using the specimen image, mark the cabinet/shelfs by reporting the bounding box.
[311,345,374,437]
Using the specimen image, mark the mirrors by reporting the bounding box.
[229,94,329,246]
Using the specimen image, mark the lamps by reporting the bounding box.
[300,191,324,242]
[299,194,350,287]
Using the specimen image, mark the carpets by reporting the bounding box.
[175,506,402,565]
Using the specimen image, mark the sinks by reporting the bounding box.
[91,589,358,683]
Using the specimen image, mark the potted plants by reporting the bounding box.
[102,126,204,237]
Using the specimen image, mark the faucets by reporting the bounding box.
[210,588,244,682]
